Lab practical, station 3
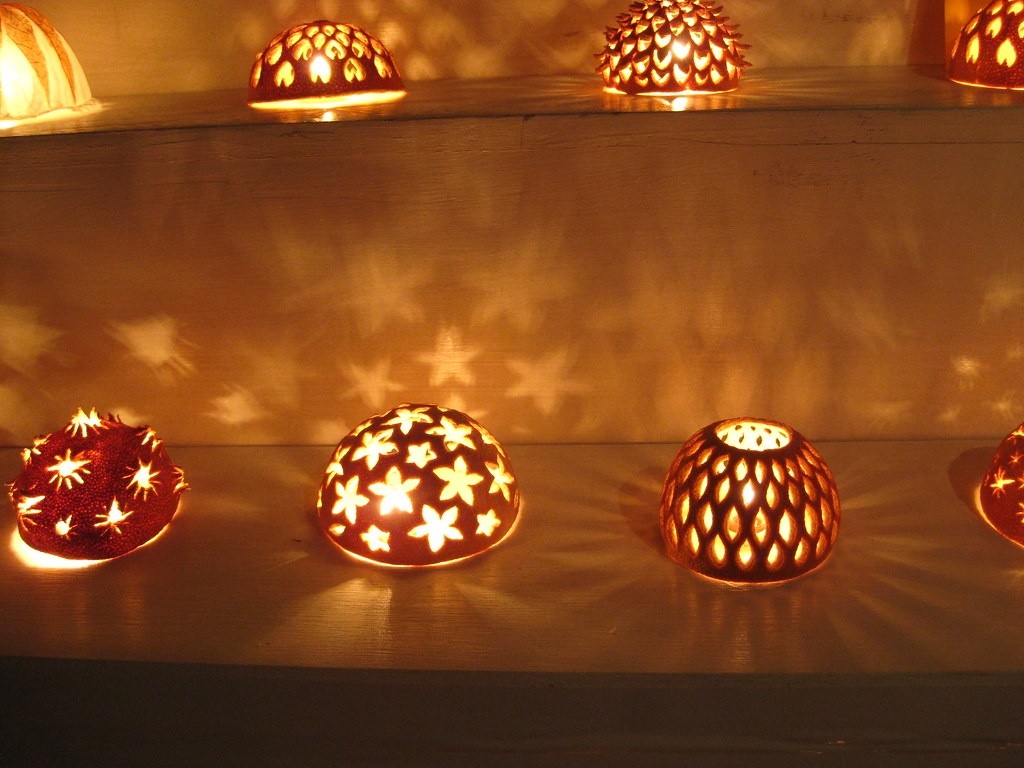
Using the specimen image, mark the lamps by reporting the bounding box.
[312,404,524,575]
[975,416,1024,562]
[655,417,844,595]
[0,2,98,133]
[945,0,1024,93]
[600,0,754,99]
[246,21,408,115]
[9,400,188,567]
